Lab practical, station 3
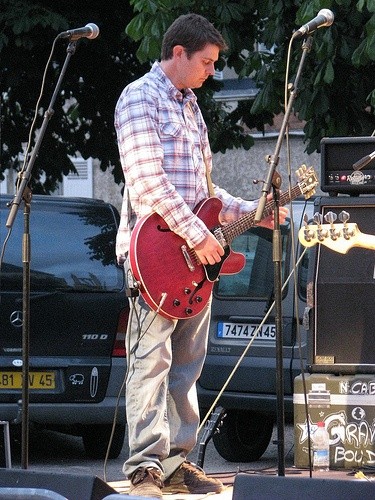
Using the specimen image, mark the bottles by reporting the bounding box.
[312,422,330,471]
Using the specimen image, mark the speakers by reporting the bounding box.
[0,469,119,500]
[308,195,375,374]
[231,472,374,500]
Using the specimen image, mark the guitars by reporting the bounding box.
[129,164,320,320]
[298,209,375,255]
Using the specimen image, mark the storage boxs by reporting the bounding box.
[292,372,375,467]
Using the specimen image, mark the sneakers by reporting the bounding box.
[161,461,224,494]
[128,467,164,498]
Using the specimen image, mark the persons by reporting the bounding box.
[115,14,289,499]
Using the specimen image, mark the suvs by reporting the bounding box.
[197,196,319,462]
[0,193,132,460]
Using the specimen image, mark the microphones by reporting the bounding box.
[60,22,100,39]
[293,8,334,39]
